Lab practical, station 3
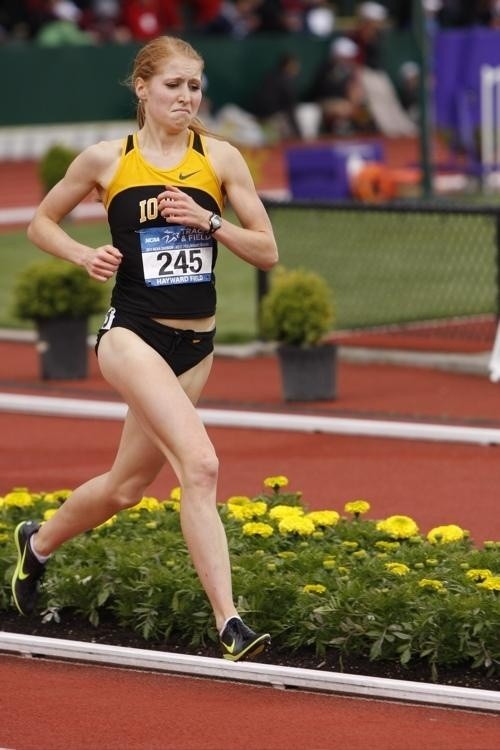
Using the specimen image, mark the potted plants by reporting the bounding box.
[13,258,107,378]
[260,268,338,402]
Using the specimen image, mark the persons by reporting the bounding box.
[0,0,267,47]
[261,3,421,142]
[11,36,279,661]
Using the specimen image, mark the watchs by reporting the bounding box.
[207,212,221,239]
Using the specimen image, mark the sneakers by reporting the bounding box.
[11,519,53,619]
[216,617,272,662]
